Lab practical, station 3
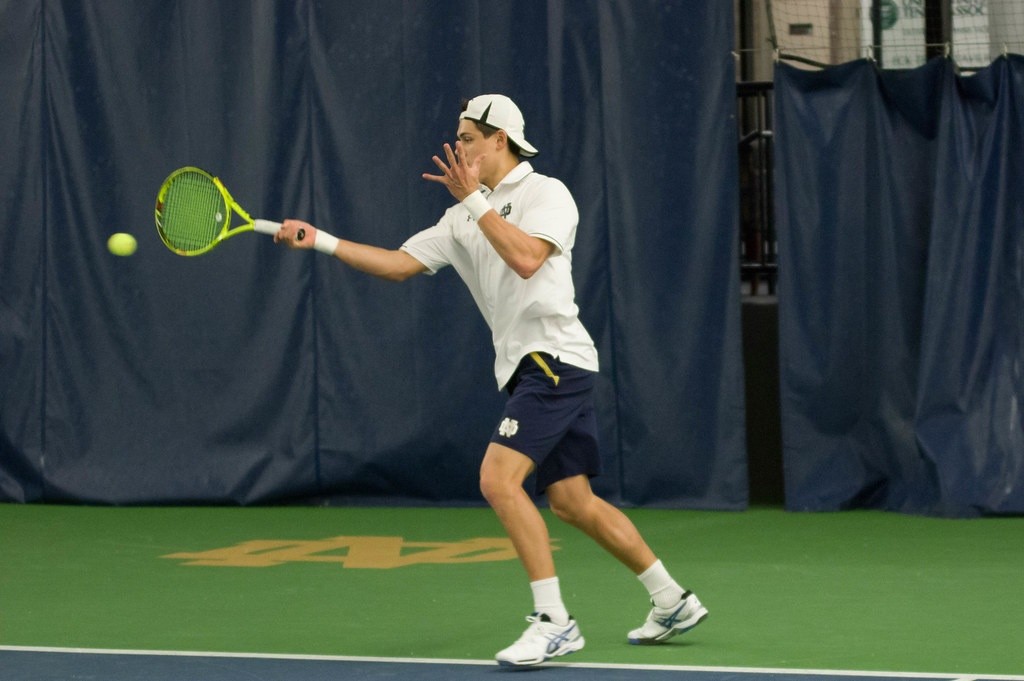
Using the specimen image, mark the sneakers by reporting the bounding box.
[627,590,710,644]
[496,608,586,667]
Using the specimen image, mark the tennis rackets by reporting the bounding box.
[155,166,306,256]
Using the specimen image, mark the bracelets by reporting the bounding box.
[460,189,493,222]
[314,229,339,257]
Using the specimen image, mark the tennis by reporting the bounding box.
[107,232,137,256]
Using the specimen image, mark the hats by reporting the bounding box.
[459,95,538,157]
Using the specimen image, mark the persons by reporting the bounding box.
[273,92,711,665]
